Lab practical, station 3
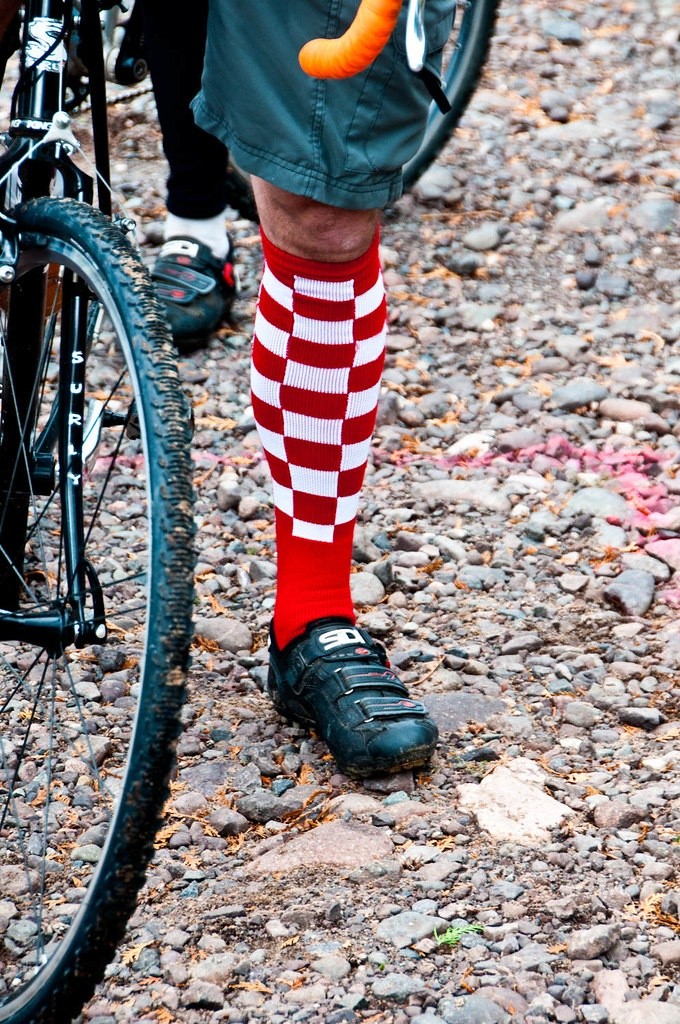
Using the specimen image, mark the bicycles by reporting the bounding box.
[0,0,199,1024]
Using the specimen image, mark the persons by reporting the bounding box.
[147,0,235,353]
[186,0,470,774]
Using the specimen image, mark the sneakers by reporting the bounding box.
[267,615,439,775]
[149,232,238,347]
